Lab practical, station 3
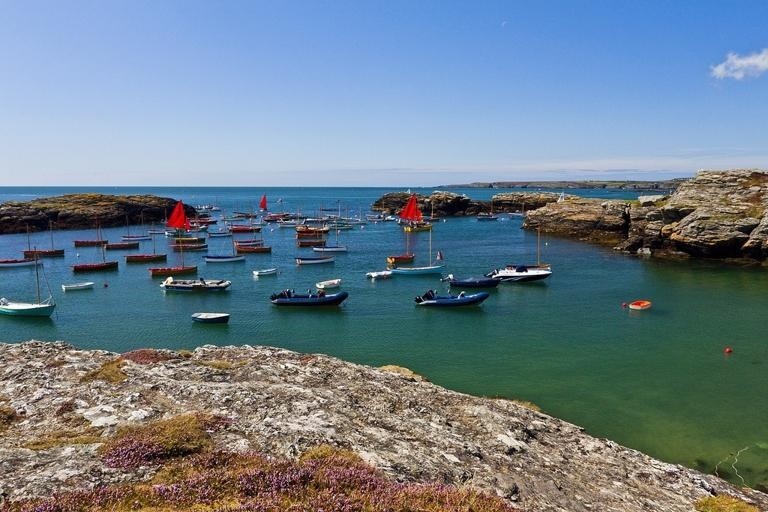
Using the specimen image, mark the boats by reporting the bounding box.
[629,300,653,311]
[160,276,232,291]
[70,192,366,276]
[191,312,230,323]
[270,278,348,306]
[0,246,56,317]
[366,192,552,306]
[0,217,64,268]
[61,281,95,291]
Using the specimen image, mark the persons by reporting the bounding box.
[284,289,291,298]
[318,289,325,298]
